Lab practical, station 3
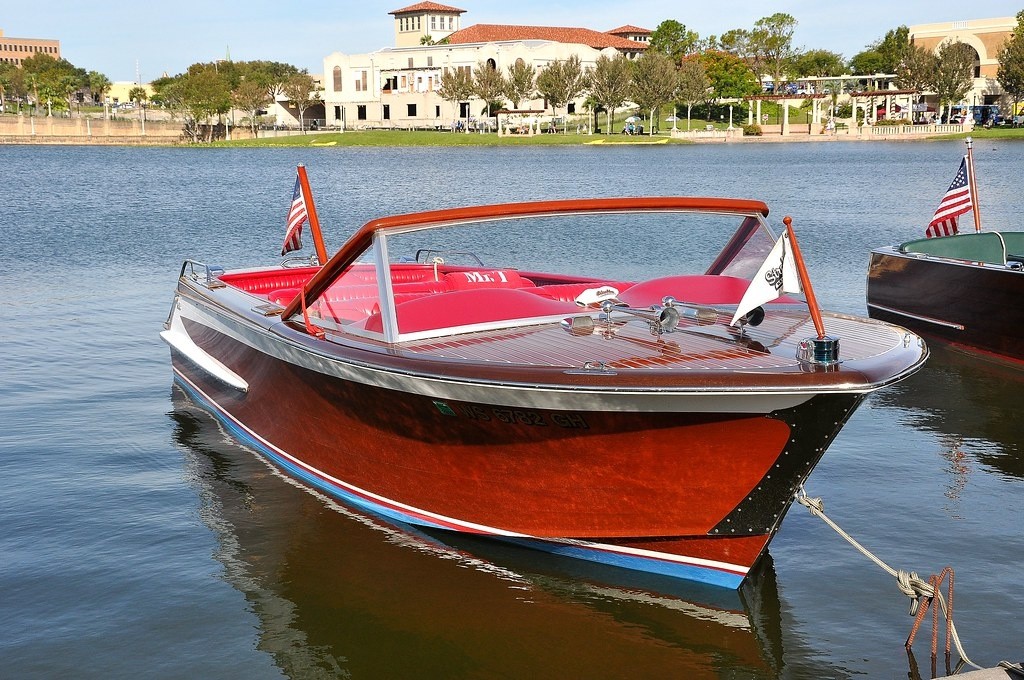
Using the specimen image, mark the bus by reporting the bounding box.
[944,104,1004,125]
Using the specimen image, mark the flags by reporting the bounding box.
[925,155,975,237]
[730,230,802,326]
[279,174,307,255]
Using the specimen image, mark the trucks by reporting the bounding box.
[1003,102,1024,124]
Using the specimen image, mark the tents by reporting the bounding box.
[901,103,937,125]
[877,102,901,115]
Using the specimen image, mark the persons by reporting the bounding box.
[621,122,643,135]
[509,126,529,134]
[258,122,266,130]
[310,118,319,131]
[450,116,477,133]
[824,117,835,130]
[273,119,293,131]
[570,121,586,135]
[931,113,940,120]
[547,121,557,134]
[912,114,926,125]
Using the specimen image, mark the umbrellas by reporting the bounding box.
[625,116,641,123]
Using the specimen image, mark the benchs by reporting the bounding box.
[230,269,639,331]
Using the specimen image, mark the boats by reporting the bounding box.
[865,136,1024,373]
[157,161,932,590]
